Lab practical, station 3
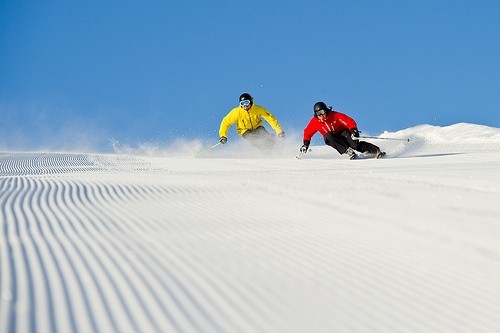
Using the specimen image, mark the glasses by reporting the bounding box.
[315,109,326,116]
[239,99,251,106]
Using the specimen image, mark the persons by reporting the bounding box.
[299,101,383,160]
[218,93,286,151]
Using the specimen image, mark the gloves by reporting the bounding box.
[300,144,309,153]
[218,137,227,144]
[353,129,359,137]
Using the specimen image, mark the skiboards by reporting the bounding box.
[348,150,387,161]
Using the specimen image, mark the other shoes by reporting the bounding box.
[374,149,385,159]
[346,147,357,160]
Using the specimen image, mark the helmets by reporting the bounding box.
[239,93,251,101]
[314,102,327,112]
